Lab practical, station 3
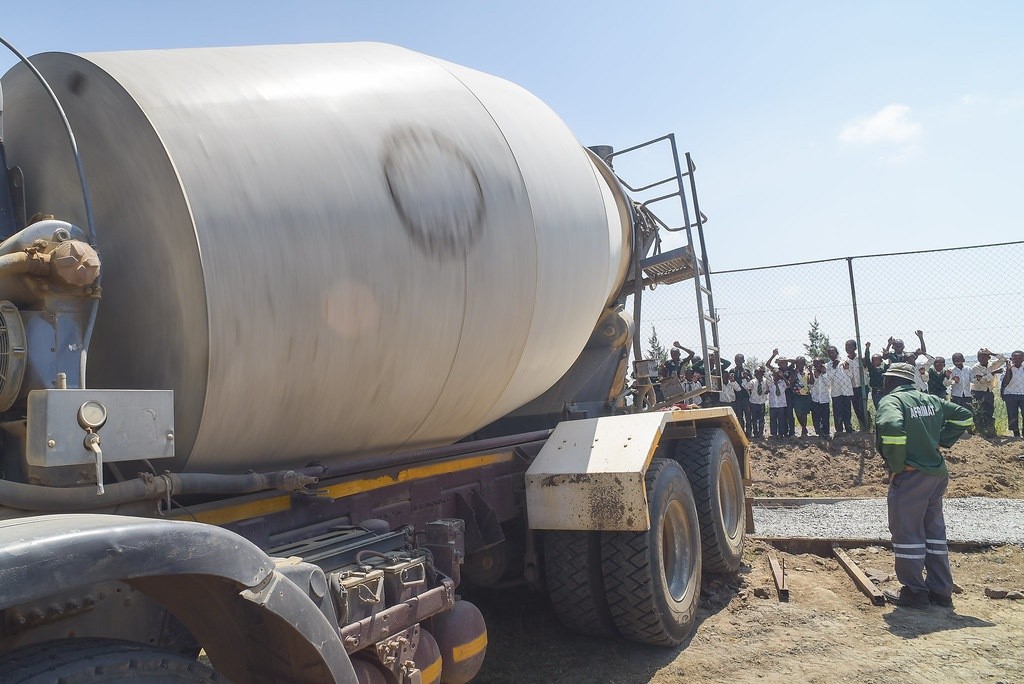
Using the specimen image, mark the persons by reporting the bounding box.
[948,352,983,434]
[825,345,855,433]
[874,362,974,608]
[1000,349,1024,440]
[808,357,830,437]
[865,341,897,411]
[903,348,935,393]
[970,347,1006,438]
[918,357,952,400]
[882,328,927,363]
[655,339,811,439]
[843,340,871,432]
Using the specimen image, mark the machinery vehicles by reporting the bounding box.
[1,34,757,684]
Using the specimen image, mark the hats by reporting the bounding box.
[775,357,790,363]
[881,362,916,384]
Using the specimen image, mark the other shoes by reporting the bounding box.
[1014,430,1019,438]
[884,586,930,608]
[924,580,953,606]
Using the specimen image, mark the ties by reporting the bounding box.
[688,384,693,405]
[757,380,763,396]
[774,383,781,396]
